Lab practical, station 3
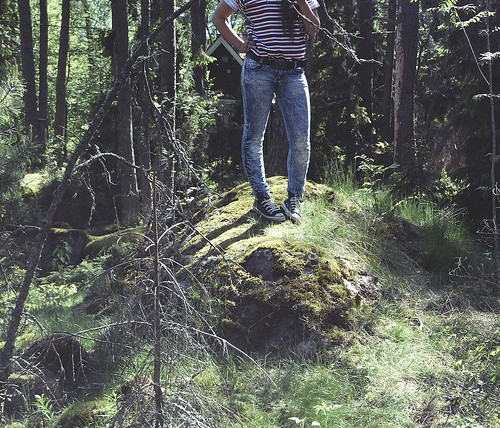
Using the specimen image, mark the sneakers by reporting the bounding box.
[282,196,303,220]
[252,197,287,221]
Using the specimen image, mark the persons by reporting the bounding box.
[212,0,321,222]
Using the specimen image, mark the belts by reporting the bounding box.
[248,52,309,70]
